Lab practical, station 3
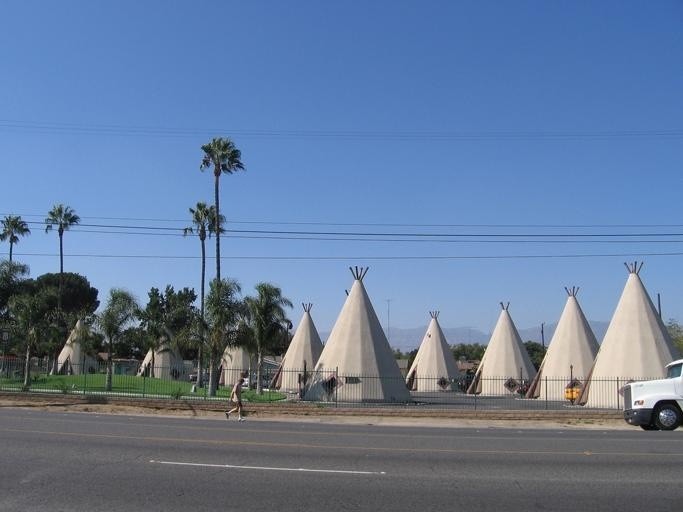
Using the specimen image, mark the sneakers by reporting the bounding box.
[225,412,230,419]
[238,418,246,422]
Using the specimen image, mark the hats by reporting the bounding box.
[237,379,244,382]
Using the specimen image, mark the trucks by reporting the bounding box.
[616,357,683,432]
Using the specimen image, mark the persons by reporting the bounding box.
[224,379,247,422]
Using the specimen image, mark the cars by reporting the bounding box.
[458,374,475,392]
[187,366,275,390]
[564,385,582,400]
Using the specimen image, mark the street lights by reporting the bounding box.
[384,298,397,339]
[541,321,547,349]
